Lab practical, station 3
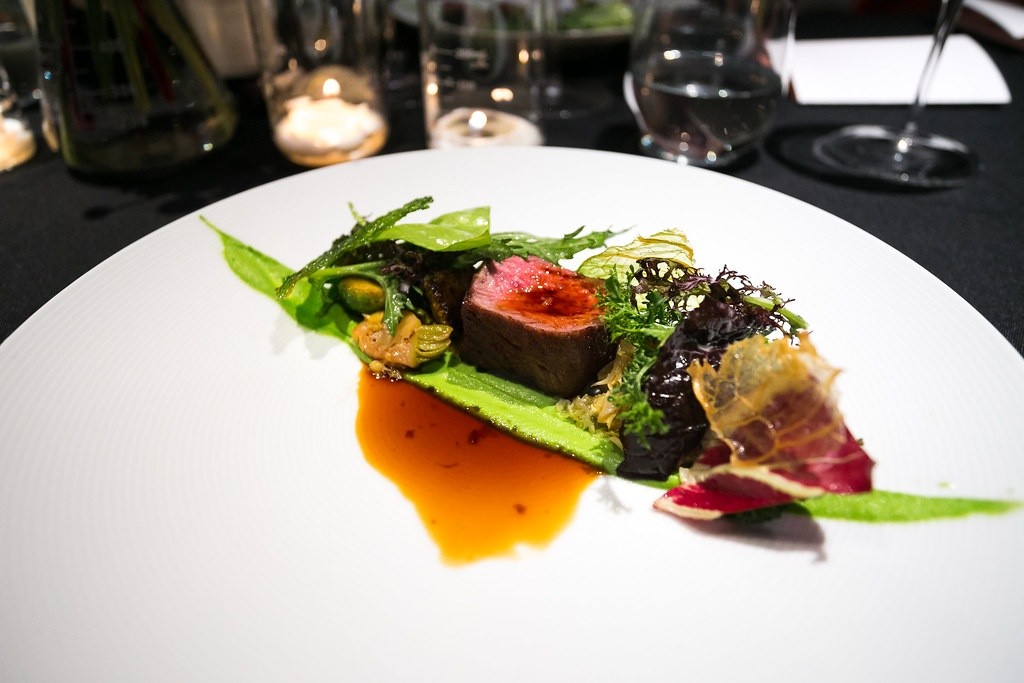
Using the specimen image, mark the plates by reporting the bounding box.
[388,0,637,47]
[0,145,1024,683]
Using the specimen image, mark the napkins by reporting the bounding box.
[763,32,1012,105]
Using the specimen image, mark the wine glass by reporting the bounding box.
[810,0,988,187]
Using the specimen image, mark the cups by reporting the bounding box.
[0,61,37,176]
[245,0,389,167]
[420,0,547,147]
[34,0,236,178]
[618,0,798,167]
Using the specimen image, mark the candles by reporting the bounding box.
[275,78,383,157]
[435,108,544,147]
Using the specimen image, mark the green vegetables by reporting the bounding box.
[200,197,1024,523]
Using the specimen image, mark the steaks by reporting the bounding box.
[457,252,619,401]
